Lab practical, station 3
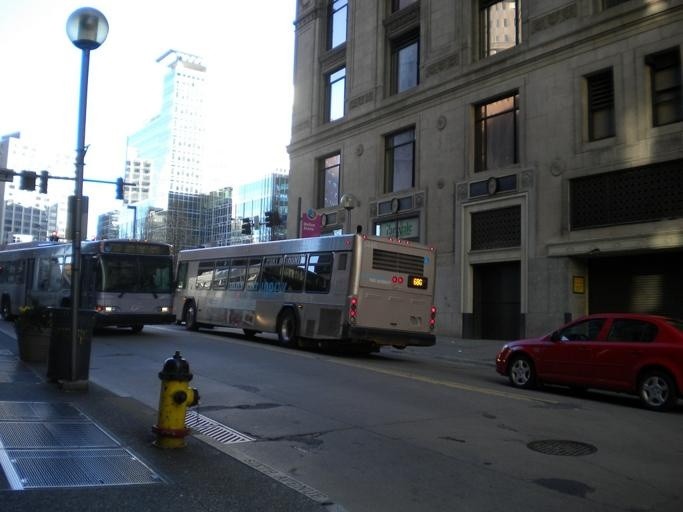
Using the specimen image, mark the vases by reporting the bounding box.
[17,328,51,363]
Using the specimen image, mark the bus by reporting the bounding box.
[0,237,173,333]
[173,232,438,355]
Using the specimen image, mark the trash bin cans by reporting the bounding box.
[46,309,95,384]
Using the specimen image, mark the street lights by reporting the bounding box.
[339,192,358,234]
[62,7,109,394]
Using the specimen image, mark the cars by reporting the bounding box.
[494,311,683,410]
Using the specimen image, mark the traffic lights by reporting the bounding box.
[241,218,252,234]
[265,211,274,227]
[49,230,58,241]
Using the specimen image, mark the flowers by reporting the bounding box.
[11,293,56,333]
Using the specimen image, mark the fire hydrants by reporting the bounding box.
[152,350,202,451]
